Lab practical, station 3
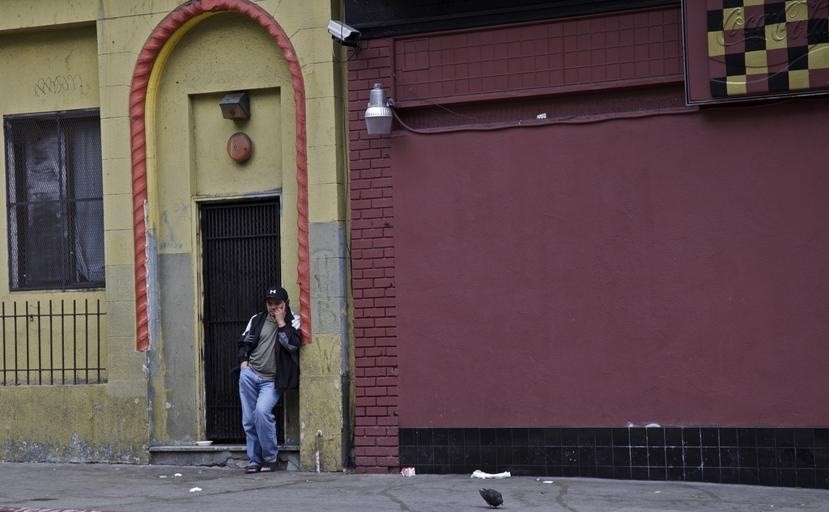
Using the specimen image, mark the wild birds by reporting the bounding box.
[478,487,504,509]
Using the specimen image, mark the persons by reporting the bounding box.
[237,286,304,474]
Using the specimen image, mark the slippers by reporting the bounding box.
[246,463,260,474]
[261,454,279,472]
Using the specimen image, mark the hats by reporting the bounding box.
[264,287,288,300]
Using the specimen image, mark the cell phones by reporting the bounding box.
[285,299,290,312]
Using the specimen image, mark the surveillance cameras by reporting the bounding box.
[328,20,362,47]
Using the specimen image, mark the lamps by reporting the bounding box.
[363,80,396,135]
[220,93,250,120]
